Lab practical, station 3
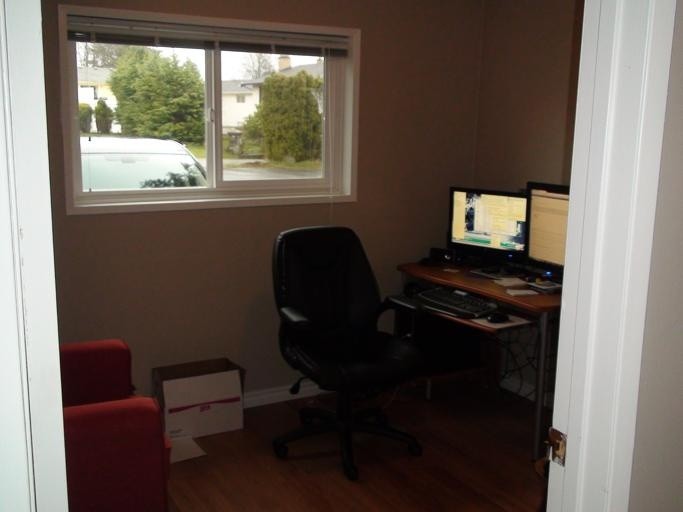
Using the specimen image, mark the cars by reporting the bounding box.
[79,134,209,192]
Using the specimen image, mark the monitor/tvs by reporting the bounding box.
[528,183,569,293]
[449,185,527,282]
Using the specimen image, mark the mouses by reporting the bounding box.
[487,310,511,324]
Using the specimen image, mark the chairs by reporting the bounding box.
[272,225,423,479]
[58,338,165,511]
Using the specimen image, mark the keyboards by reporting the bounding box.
[417,284,502,318]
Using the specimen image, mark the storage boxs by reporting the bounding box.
[151,357,247,441]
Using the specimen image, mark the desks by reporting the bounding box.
[395,257,560,463]
